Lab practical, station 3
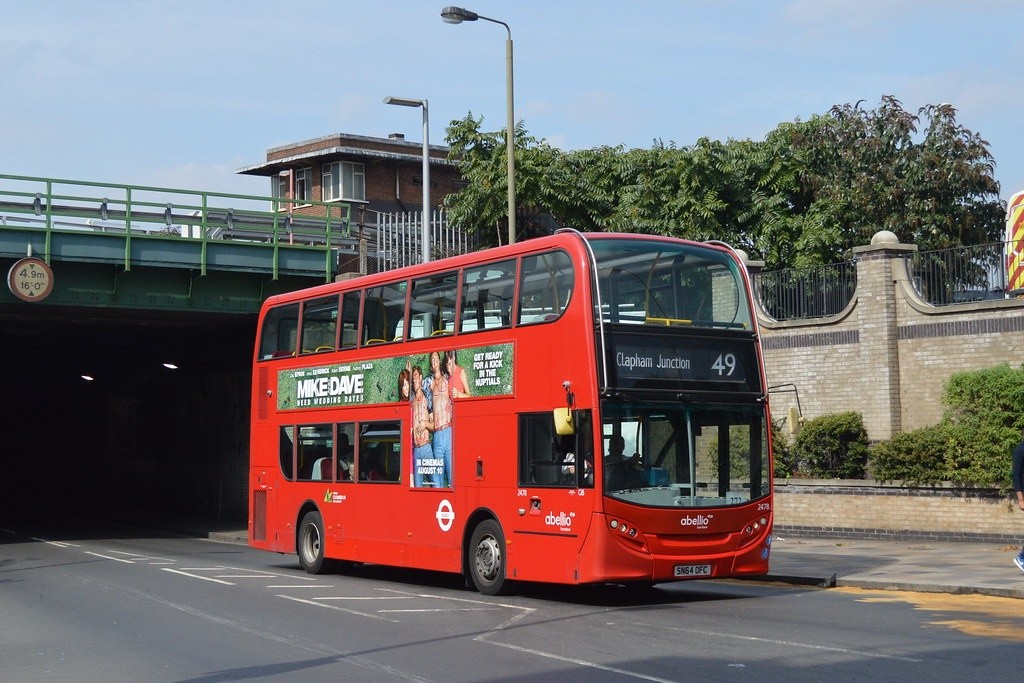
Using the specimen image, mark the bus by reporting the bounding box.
[247,228,806,594]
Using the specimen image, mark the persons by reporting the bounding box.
[339,432,400,481]
[396,348,474,488]
[562,430,642,483]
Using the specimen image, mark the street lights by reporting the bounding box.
[279,168,293,199]
[441,6,515,216]
[383,96,430,222]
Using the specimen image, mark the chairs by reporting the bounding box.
[270,329,458,359]
